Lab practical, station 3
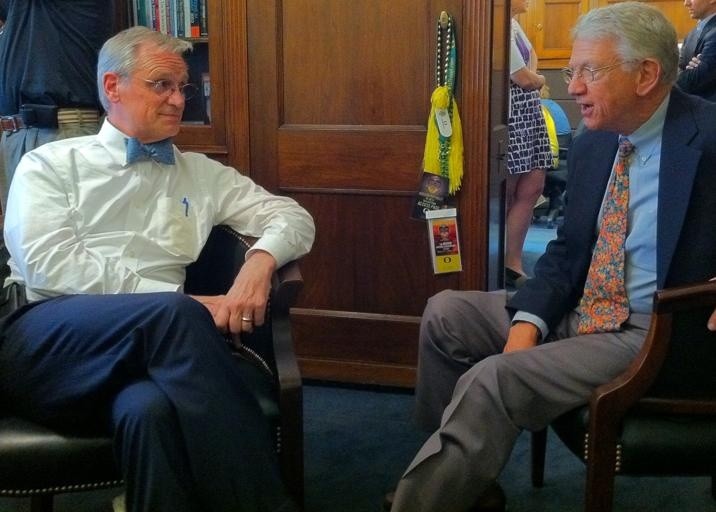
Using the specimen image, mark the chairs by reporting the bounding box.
[531,98,573,229]
[1,223,306,509]
[529,276,716,509]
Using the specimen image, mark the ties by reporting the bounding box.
[578,140,632,334]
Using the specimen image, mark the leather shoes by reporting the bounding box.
[505,266,528,289]
[385,481,505,511]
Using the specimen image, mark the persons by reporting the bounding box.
[1,24,316,512]
[384,2,715,510]
[504,0,557,290]
[676,0,715,103]
[0,0,108,225]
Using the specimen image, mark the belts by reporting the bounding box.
[1,116,25,132]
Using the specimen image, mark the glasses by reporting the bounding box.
[133,77,199,101]
[560,60,635,85]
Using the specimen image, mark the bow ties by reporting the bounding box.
[127,137,175,166]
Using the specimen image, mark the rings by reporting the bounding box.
[241,317,253,321]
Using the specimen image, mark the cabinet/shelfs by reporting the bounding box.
[116,1,226,153]
